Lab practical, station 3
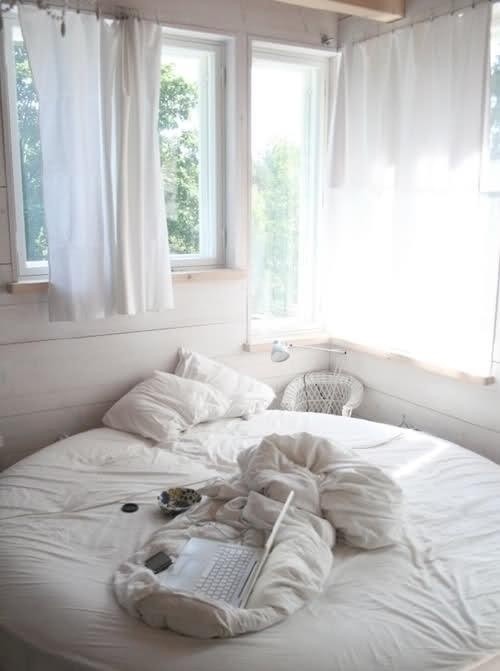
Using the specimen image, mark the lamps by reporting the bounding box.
[268,338,348,364]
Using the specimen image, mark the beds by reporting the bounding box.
[3,409,500,671]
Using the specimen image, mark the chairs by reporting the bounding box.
[281,370,365,418]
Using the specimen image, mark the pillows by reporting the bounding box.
[105,348,275,441]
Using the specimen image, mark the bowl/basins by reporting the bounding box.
[157,488,201,518]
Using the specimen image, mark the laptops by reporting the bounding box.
[161,489,294,609]
